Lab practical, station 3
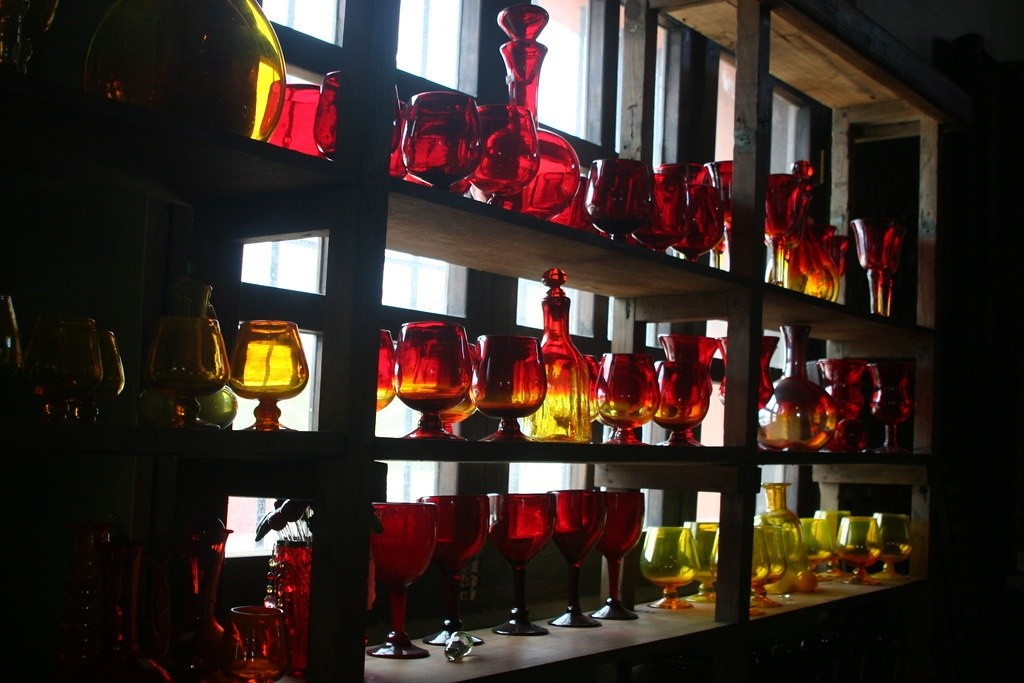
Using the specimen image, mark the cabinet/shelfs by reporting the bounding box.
[0,0,963,683]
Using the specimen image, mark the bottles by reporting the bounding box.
[470,2,581,222]
[524,268,592,443]
[755,480,807,594]
[755,321,837,453]
[769,159,840,306]
[80,0,285,146]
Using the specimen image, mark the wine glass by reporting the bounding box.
[0,0,916,682]
